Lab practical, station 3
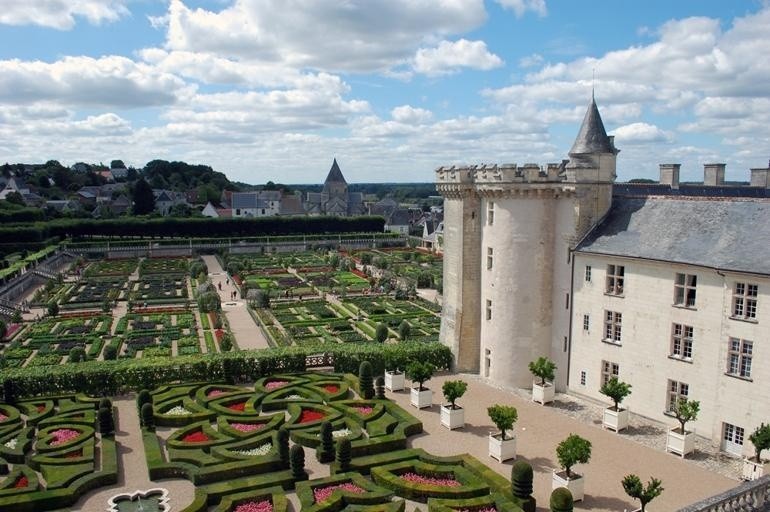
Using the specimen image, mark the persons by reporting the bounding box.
[217,279,239,299]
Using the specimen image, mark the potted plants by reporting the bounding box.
[741,422,770,485]
[619,473,667,512]
[551,432,593,507]
[599,376,633,432]
[663,393,703,462]
[439,379,469,429]
[527,356,560,406]
[483,402,520,464]
[382,345,408,391]
[406,361,437,410]
